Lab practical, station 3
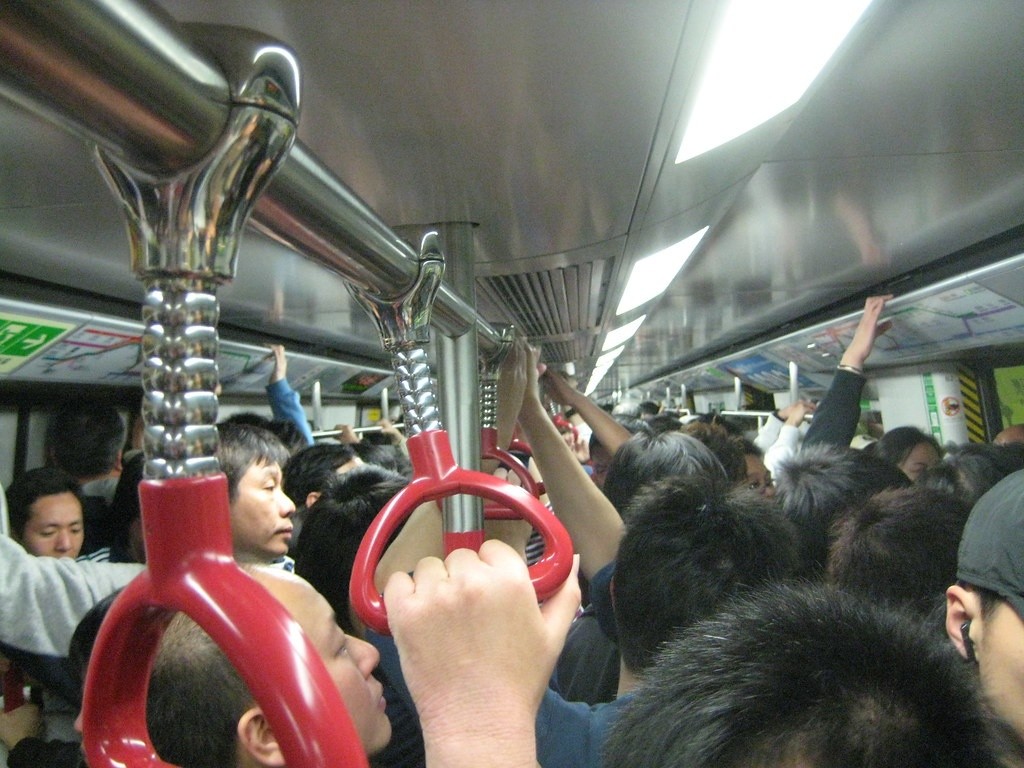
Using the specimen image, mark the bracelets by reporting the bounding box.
[771,409,786,421]
[837,364,864,377]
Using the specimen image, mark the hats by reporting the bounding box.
[956,469,1024,622]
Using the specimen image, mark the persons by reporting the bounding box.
[0,292,1024,768]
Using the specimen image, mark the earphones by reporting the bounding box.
[962,619,977,664]
